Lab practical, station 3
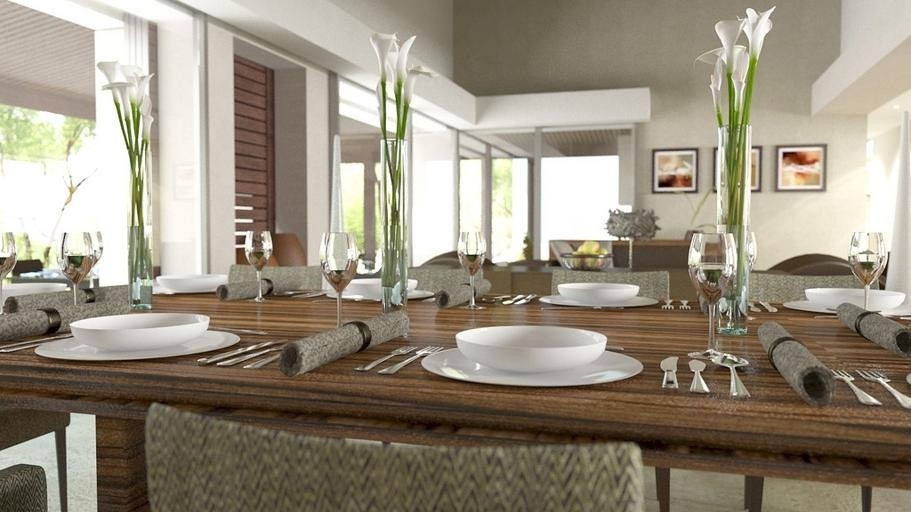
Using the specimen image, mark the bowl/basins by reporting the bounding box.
[804,285,904,312]
[557,282,639,303]
[157,273,228,290]
[71,311,210,350]
[456,324,608,373]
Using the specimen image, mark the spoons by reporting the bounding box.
[715,353,753,399]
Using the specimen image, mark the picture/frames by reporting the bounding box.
[649,146,700,195]
[709,144,764,195]
[772,143,830,193]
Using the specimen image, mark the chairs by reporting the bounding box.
[146,407,639,512]
[1,257,70,511]
[328,238,892,292]
[551,267,874,511]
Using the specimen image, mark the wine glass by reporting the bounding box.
[0,231,19,312]
[89,231,103,288]
[850,230,893,309]
[56,232,94,307]
[687,231,759,361]
[243,229,275,301]
[458,231,494,311]
[321,231,361,327]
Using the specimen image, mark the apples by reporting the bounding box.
[572,241,608,268]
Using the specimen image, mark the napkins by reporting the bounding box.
[218,276,299,303]
[837,302,911,359]
[434,278,492,309]
[279,313,410,376]
[3,283,153,315]
[0,300,132,342]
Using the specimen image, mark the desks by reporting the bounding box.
[0,294,911,512]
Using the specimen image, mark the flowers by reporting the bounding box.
[693,4,780,324]
[368,26,440,276]
[91,52,158,287]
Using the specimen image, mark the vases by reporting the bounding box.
[379,138,408,313]
[125,165,155,311]
[714,121,755,339]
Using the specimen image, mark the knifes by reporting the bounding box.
[761,301,780,313]
[689,358,711,394]
[748,301,760,314]
[479,294,537,306]
[659,354,681,388]
[199,337,290,369]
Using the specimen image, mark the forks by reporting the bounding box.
[661,297,676,309]
[680,300,691,312]
[831,369,882,406]
[353,344,416,372]
[855,365,911,407]
[383,344,444,376]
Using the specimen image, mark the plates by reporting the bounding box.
[423,347,645,387]
[539,295,658,309]
[33,330,239,362]
[784,298,910,318]
